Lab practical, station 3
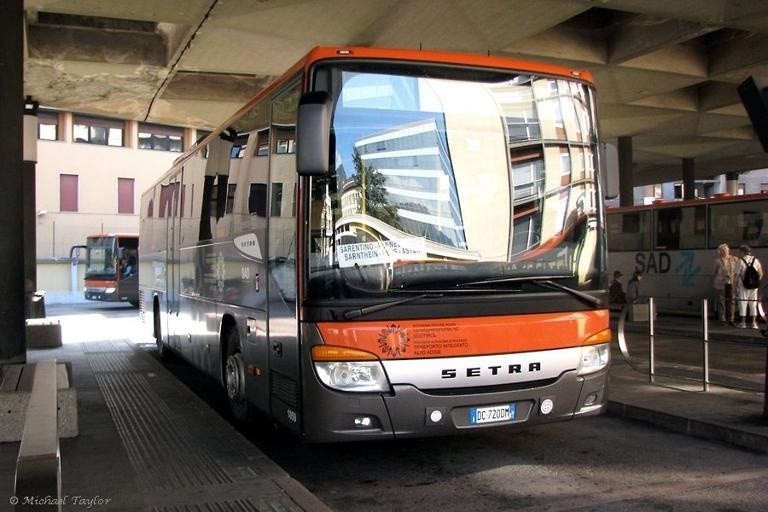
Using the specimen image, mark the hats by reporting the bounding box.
[613,270,624,277]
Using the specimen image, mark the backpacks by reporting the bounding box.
[739,256,760,289]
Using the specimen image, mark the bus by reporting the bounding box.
[71,45,768,443]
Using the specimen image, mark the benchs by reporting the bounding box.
[0,361,80,443]
[609,303,656,322]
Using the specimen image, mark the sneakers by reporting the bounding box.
[751,323,758,329]
[737,322,747,329]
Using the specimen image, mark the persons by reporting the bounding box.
[710,242,742,328]
[608,269,627,303]
[625,269,659,321]
[117,258,134,278]
[731,243,763,330]
